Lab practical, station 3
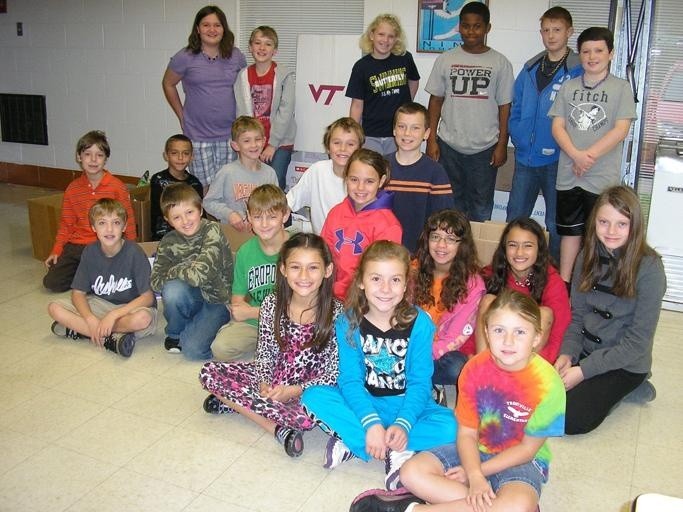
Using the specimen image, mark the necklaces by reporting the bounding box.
[541,48,570,78]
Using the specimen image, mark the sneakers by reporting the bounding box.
[104,332,136,357]
[275,425,304,457]
[348,488,427,512]
[323,436,354,469]
[51,320,90,341]
[164,337,182,354]
[623,381,656,404]
[384,448,416,492]
[203,393,240,415]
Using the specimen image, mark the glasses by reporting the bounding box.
[429,234,463,244]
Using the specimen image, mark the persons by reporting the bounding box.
[349,288,567,510]
[505,7,584,264]
[554,185,667,435]
[233,25,296,196]
[198,232,345,458]
[378,102,456,253]
[208,183,297,360]
[46,198,157,356]
[286,118,364,237]
[402,210,486,405]
[298,238,458,491]
[456,217,571,364]
[202,116,279,235]
[544,27,639,295]
[149,135,204,239]
[147,182,234,360]
[42,129,137,293]
[424,3,514,221]
[320,149,402,303]
[343,14,420,156]
[161,6,248,219]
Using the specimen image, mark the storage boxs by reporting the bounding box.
[469,219,555,272]
[25,180,150,264]
[126,183,210,242]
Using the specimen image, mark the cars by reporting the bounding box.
[641,54,683,172]
